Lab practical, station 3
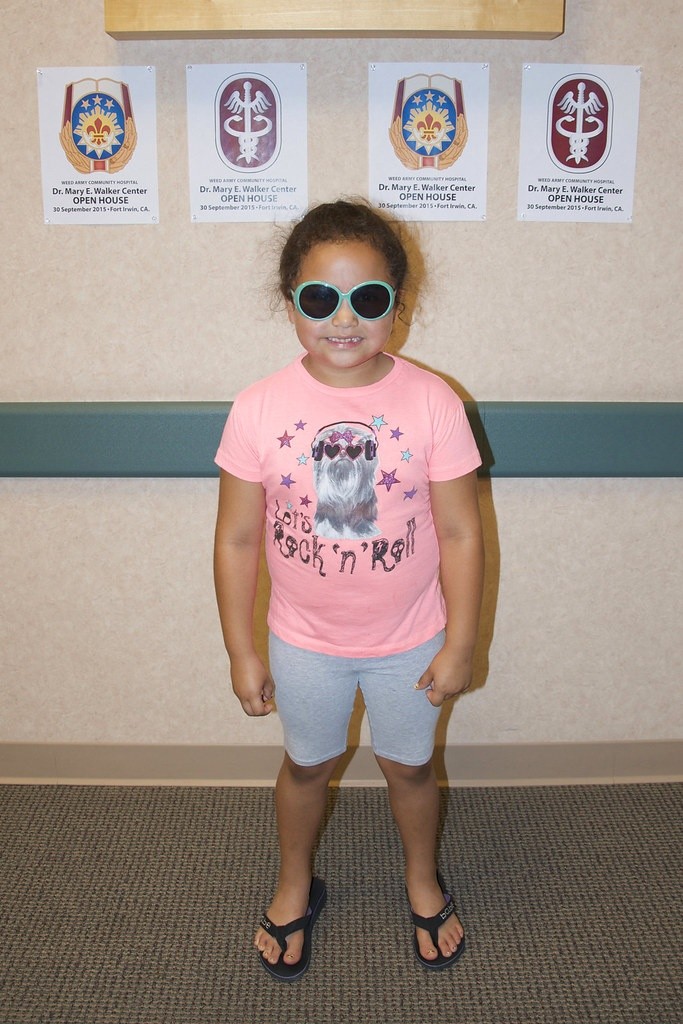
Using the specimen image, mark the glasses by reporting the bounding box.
[283,280,398,320]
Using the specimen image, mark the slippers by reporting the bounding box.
[255,874,325,983]
[403,870,467,974]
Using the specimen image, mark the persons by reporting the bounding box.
[215,202,485,979]
